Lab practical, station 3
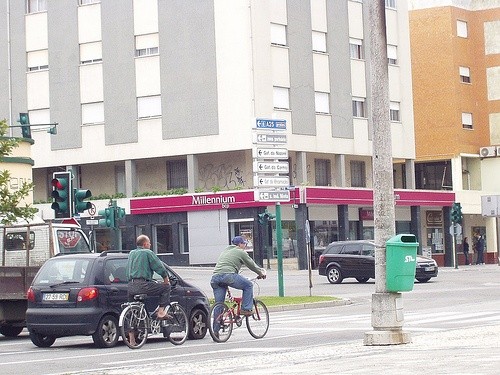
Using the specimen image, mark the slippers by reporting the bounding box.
[129,342,139,348]
[156,313,173,320]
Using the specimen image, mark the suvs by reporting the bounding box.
[24,249,211,348]
[318,239,439,283]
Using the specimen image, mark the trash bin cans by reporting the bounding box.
[384,233,419,292]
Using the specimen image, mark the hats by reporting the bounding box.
[232,236,248,244]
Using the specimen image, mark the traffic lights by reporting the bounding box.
[73,187,92,214]
[450,210,458,221]
[453,203,463,222]
[98,208,112,228]
[117,207,125,219]
[15,112,31,139]
[50,127,57,135]
[51,172,72,219]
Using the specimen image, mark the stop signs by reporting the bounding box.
[57,218,81,248]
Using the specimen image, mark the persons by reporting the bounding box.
[463,236,471,265]
[473,232,486,265]
[210,236,267,342]
[127,234,173,349]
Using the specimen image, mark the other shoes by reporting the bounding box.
[240,309,255,315]
[213,332,219,341]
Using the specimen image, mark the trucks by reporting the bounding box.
[0,219,98,338]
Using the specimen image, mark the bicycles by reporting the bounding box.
[118,276,190,349]
[207,274,270,344]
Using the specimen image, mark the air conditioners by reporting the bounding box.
[480,146,496,157]
[496,145,500,156]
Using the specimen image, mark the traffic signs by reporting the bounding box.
[254,161,290,173]
[252,132,287,144]
[256,176,290,187]
[255,191,290,202]
[253,118,287,130]
[254,147,289,159]
